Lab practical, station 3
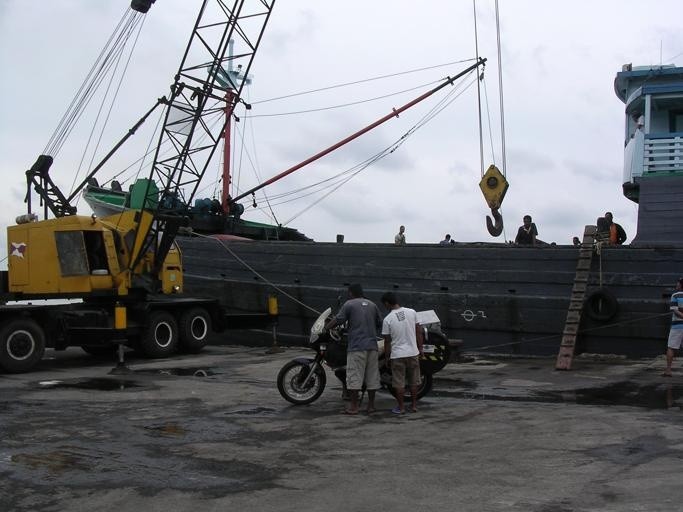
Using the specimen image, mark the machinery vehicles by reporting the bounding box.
[0,0,509,374]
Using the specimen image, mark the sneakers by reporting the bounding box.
[392,405,419,414]
[339,406,376,416]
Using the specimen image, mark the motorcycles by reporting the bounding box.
[277,307,450,405]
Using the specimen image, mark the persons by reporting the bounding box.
[604,212,627,246]
[325,283,383,415]
[381,292,424,414]
[658,277,683,377]
[440,234,451,244]
[515,216,538,247]
[394,226,406,244]
[573,237,579,245]
